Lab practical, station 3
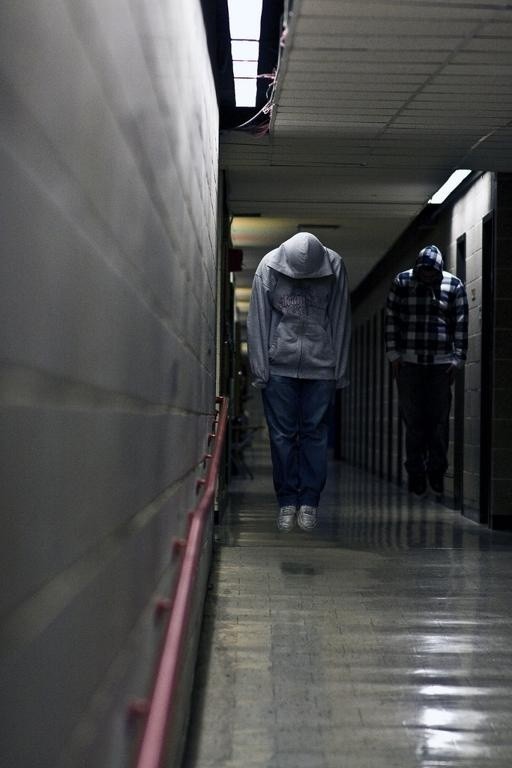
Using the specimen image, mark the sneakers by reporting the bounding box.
[428,465,446,494]
[408,468,427,495]
[297,502,320,532]
[275,504,298,533]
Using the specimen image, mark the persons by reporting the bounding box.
[247,231,351,533]
[386,244,469,499]
